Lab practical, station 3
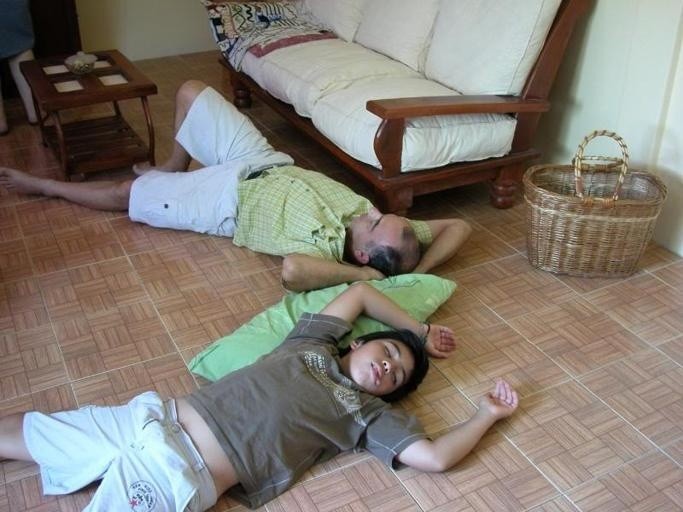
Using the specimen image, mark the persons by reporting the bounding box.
[0,0,40,133]
[0,79,473,292]
[0,282,520,512]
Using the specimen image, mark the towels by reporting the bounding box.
[201,0,338,71]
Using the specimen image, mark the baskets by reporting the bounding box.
[524,131,667,279]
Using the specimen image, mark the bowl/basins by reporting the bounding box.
[63,51,96,75]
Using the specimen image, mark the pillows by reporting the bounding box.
[187,273,459,384]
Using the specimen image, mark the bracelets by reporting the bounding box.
[422,323,430,345]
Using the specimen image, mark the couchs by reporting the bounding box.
[202,0,591,217]
